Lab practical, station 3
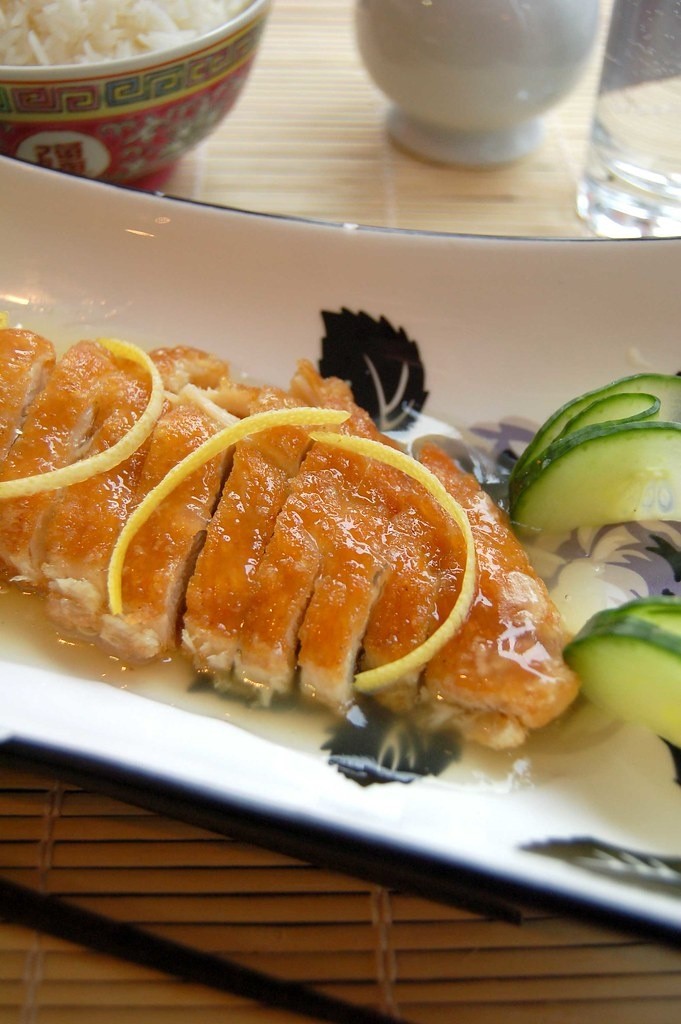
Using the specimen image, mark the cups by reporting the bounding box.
[352,0,605,169]
[574,0,681,240]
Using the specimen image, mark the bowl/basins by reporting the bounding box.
[0,0,274,193]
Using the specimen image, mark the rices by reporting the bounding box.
[0,0,257,67]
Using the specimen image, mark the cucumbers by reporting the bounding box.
[506,373,681,751]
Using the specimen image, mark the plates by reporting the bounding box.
[0,154,681,929]
